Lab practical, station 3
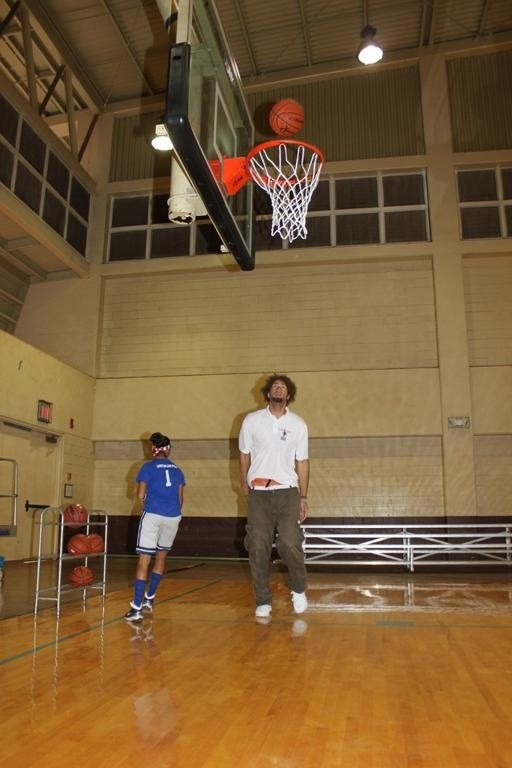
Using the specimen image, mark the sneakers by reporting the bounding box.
[123,602,144,622]
[254,604,272,618]
[290,590,308,614]
[142,591,156,609]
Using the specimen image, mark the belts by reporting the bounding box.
[252,485,296,491]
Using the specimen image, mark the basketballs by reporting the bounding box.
[69,565,94,588]
[269,99,305,137]
[68,533,104,555]
[64,504,88,529]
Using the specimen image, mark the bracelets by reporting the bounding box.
[300,496,308,499]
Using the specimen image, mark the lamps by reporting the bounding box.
[356,0,384,65]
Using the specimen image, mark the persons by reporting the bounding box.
[126,617,180,747]
[124,430,187,625]
[237,372,312,619]
